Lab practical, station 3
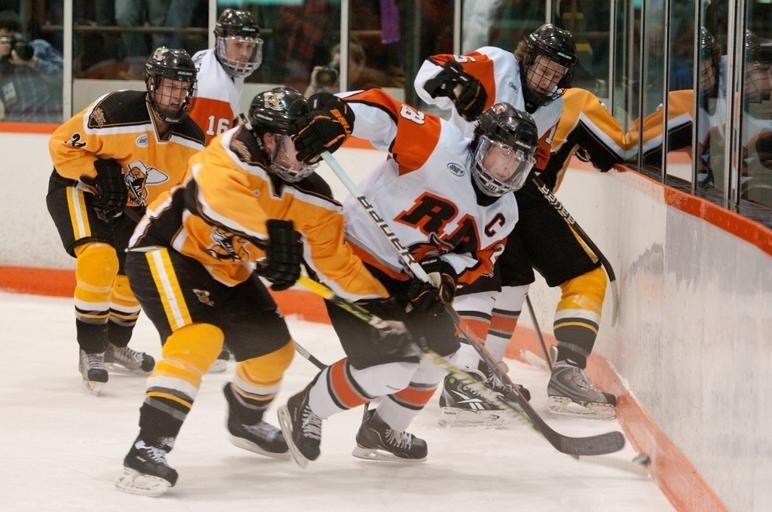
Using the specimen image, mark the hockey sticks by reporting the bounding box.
[452,83,618,325]
[248,259,652,480]
[321,150,626,456]
[525,290,558,373]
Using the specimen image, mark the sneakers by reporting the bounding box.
[287,385,322,460]
[125,436,178,484]
[229,418,288,453]
[78,350,109,382]
[359,410,428,458]
[549,345,616,405]
[105,347,155,373]
[478,360,531,402]
[440,369,511,409]
[218,347,230,360]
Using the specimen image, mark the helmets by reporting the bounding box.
[516,23,576,105]
[248,87,320,184]
[744,30,771,104]
[470,102,538,198]
[699,26,719,99]
[213,9,264,77]
[145,47,198,123]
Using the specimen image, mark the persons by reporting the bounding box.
[277,87,541,470]
[114,0,194,81]
[412,23,578,427]
[0,7,64,125]
[186,7,264,374]
[625,27,772,211]
[46,45,211,399]
[72,20,117,81]
[114,85,413,498]
[302,42,395,104]
[530,79,625,420]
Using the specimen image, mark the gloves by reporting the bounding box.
[406,258,457,317]
[257,218,303,291]
[92,158,126,220]
[291,91,356,164]
[426,59,486,121]
